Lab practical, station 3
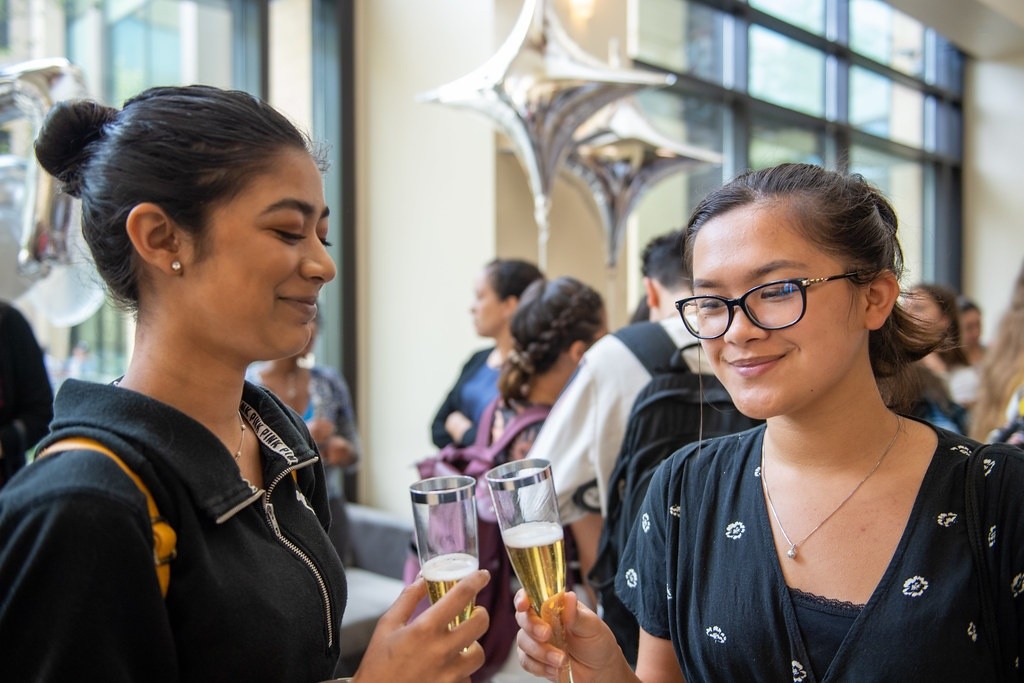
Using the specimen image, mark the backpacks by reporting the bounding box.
[588,323,766,668]
[403,400,551,683]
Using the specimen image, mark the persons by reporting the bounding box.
[521,226,765,665]
[512,162,1024,683]
[430,256,544,469]
[465,274,609,676]
[871,266,1024,449]
[247,303,363,566]
[0,83,492,683]
[0,299,53,490]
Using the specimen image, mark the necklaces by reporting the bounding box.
[109,377,248,463]
[762,413,900,557]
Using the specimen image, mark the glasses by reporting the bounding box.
[674,265,882,340]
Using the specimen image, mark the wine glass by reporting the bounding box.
[485,459,574,683]
[409,475,480,653]
